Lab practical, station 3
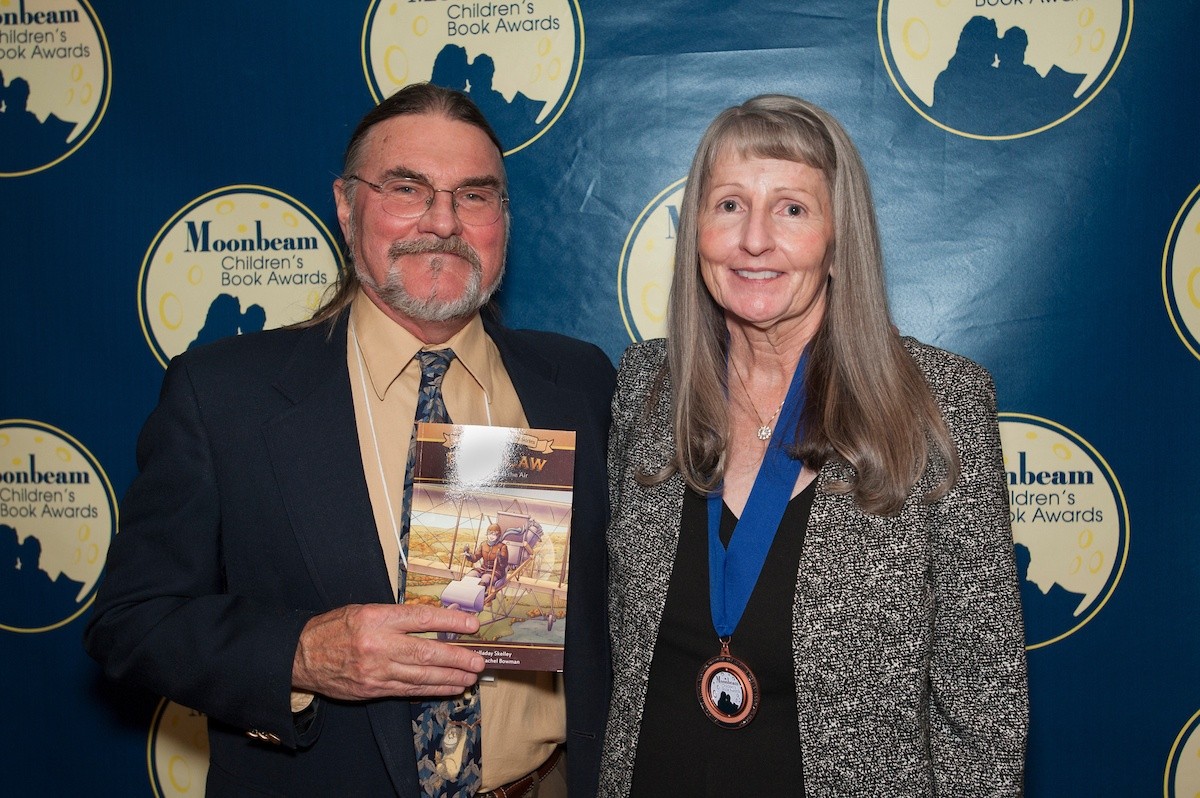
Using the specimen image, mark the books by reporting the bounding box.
[405,422,577,673]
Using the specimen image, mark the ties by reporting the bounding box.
[396,348,483,798]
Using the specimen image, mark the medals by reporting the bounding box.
[693,647,766,729]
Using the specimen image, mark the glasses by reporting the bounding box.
[345,175,508,226]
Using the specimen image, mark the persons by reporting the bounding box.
[596,96,1032,798]
[82,84,619,798]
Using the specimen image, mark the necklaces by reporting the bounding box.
[726,344,786,441]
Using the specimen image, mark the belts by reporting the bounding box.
[486,741,565,798]
